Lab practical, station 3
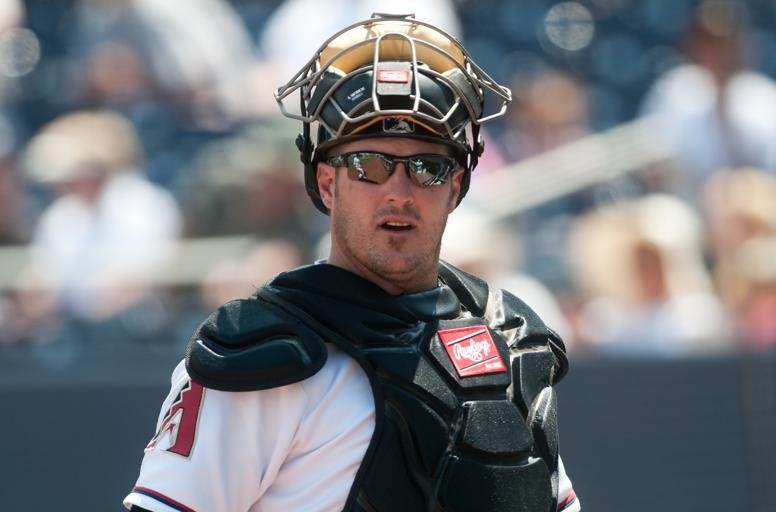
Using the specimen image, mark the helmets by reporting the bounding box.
[300,12,483,156]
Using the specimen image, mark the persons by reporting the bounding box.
[0,1,776,370]
[118,14,589,512]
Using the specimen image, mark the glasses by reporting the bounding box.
[320,150,460,189]
